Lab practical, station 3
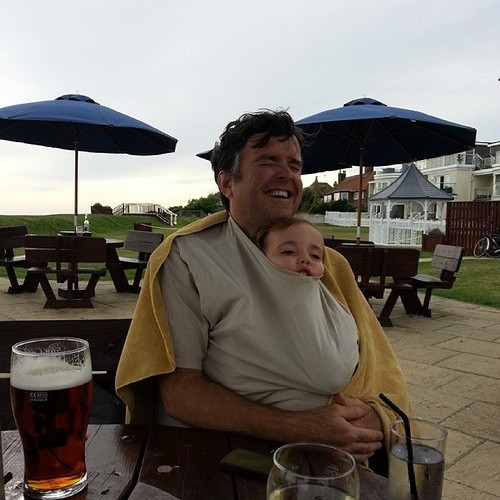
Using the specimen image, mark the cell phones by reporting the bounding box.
[220,447,300,482]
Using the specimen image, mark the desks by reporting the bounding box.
[340,243,422,315]
[19,237,130,293]
[0,423,390,500]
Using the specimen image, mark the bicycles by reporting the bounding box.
[472,223,500,259]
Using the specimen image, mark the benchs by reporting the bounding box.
[324,239,465,327]
[0,319,133,431]
[0,222,164,309]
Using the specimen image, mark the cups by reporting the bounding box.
[10,336,93,500]
[267,443,360,500]
[76,226,83,237]
[388,418,448,500]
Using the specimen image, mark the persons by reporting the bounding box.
[154,109,405,466]
[201,217,359,409]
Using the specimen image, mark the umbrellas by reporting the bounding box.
[0,95,178,232]
[195,97,476,245]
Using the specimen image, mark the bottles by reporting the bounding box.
[84,214,89,232]
